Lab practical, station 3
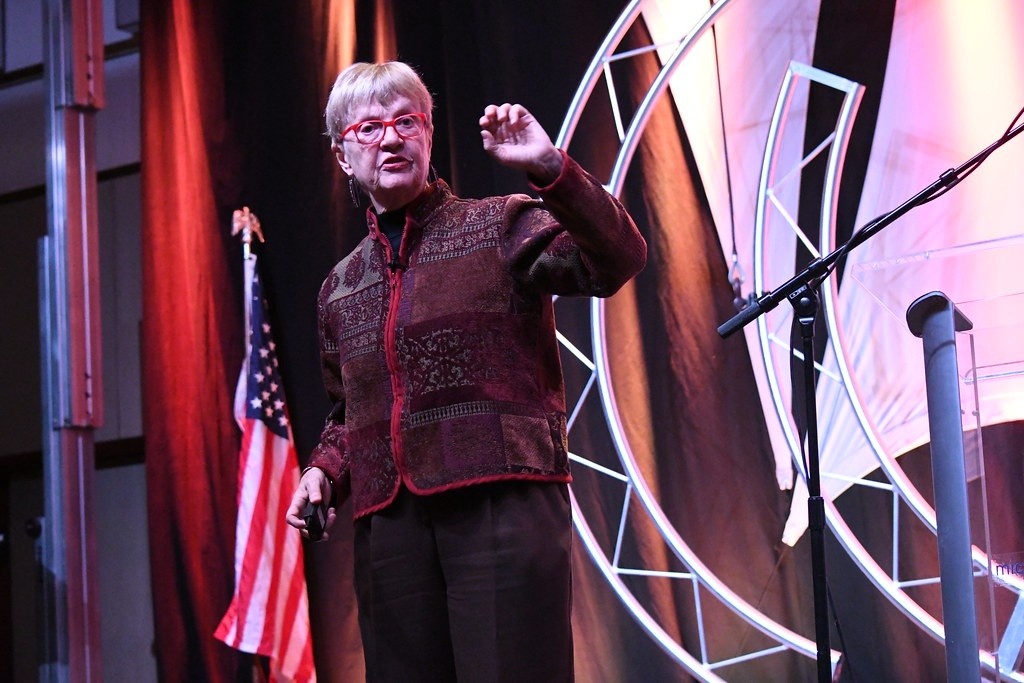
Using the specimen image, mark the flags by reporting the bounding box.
[213,251,319,683]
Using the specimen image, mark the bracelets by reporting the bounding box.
[300,466,334,487]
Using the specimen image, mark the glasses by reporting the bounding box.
[339,112,428,145]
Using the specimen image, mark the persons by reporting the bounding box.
[285,59,649,683]
